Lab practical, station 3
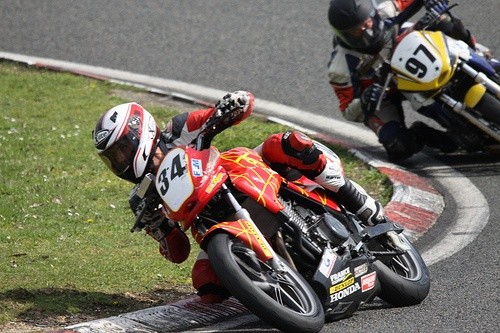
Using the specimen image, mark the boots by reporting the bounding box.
[411,122,457,152]
[314,160,384,227]
[466,27,476,51]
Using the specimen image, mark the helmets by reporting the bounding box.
[328,0,385,53]
[94,101,162,184]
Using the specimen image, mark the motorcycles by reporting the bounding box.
[129,96,431,333]
[358,0,500,164]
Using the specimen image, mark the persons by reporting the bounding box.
[326,0,500,161]
[92,90,385,304]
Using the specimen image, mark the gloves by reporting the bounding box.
[212,89,250,122]
[361,83,388,106]
[128,181,176,242]
[425,0,448,18]
